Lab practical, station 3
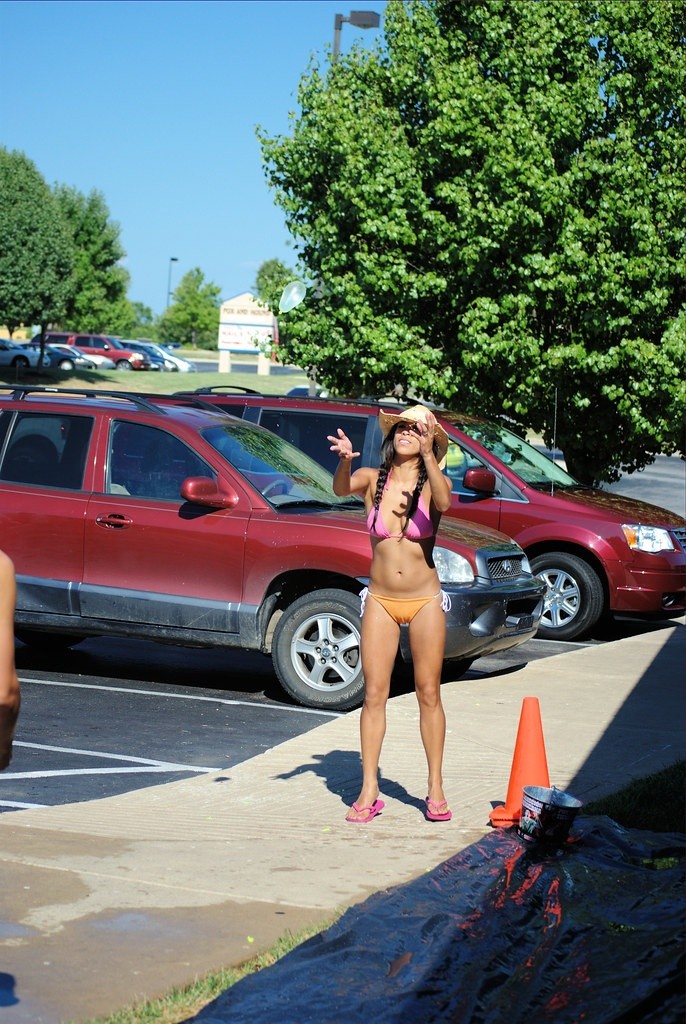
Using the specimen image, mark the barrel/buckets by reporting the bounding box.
[517,784,584,845]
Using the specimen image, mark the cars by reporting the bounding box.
[284,386,330,398]
[116,340,198,372]
[0,338,51,368]
[47,343,116,369]
[19,343,97,370]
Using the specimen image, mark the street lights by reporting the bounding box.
[166,257,178,309]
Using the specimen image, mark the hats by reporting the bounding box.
[379,404,448,463]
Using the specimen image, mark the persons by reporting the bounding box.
[327,404,453,822]
[0,548,19,773]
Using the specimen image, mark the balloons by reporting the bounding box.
[278,281,306,314]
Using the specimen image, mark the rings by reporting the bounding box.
[423,433,427,435]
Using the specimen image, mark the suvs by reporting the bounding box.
[173,385,686,641]
[30,333,152,371]
[0,383,548,711]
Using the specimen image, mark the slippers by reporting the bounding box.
[426,796,452,821]
[345,799,385,822]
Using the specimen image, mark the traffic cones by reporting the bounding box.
[489,697,550,827]
